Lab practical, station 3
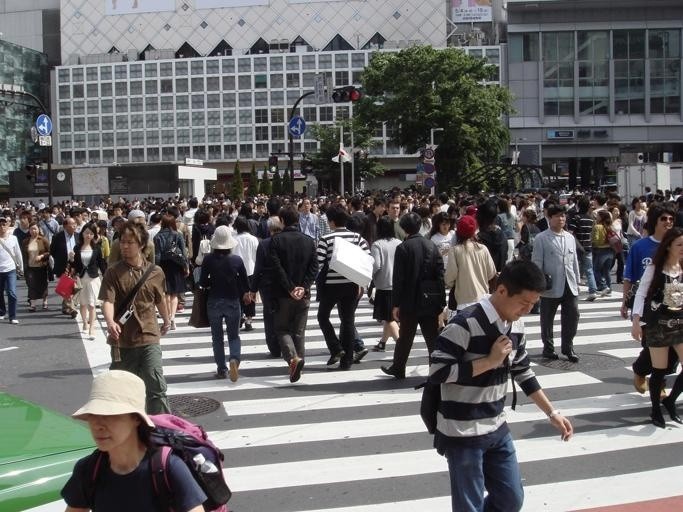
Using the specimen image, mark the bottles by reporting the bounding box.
[193,452,230,506]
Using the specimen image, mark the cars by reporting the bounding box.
[0,388,98,512]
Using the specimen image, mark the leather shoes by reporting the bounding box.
[543,347,558,359]
[561,348,580,363]
[651,403,665,429]
[634,374,649,394]
[380,365,405,378]
[662,396,683,425]
[28,305,36,312]
[43,300,48,310]
[327,349,351,370]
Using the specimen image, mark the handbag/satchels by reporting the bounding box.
[329,235,374,287]
[15,265,23,281]
[188,289,211,328]
[607,229,622,254]
[54,273,83,301]
[574,237,585,258]
[48,266,54,281]
[625,280,654,323]
[168,246,184,269]
[420,374,444,435]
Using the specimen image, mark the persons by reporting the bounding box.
[427,258,573,512]
[59,369,209,512]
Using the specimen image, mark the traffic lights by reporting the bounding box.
[333,88,360,102]
[268,155,278,175]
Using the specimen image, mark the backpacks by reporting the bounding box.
[146,411,232,512]
[195,238,212,264]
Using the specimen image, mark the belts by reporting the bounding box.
[658,319,683,329]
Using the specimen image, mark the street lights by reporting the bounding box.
[513,135,528,165]
[430,127,445,197]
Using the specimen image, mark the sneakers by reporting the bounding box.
[659,379,667,403]
[240,316,252,331]
[289,356,305,383]
[215,369,227,379]
[601,285,612,298]
[587,292,597,301]
[83,322,96,340]
[372,341,386,352]
[62,299,79,318]
[0,313,5,320]
[10,319,19,324]
[229,358,239,382]
[170,319,177,331]
[353,344,369,363]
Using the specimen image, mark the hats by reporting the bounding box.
[0,216,8,222]
[210,224,238,250]
[455,215,477,235]
[128,209,145,221]
[71,369,155,429]
